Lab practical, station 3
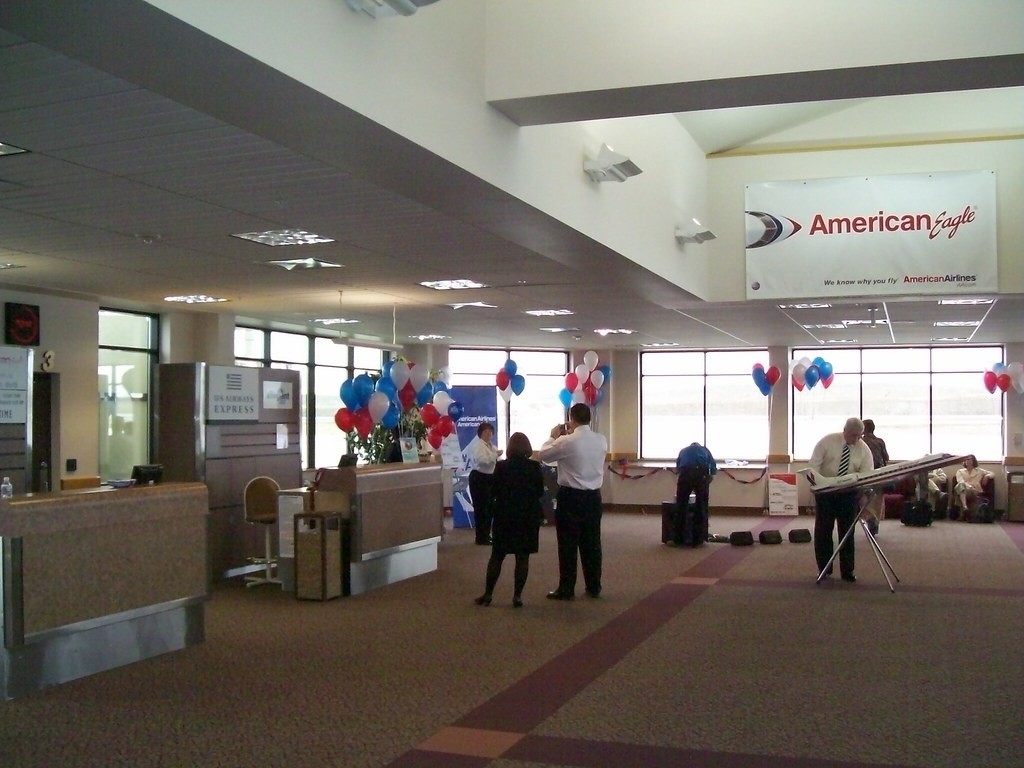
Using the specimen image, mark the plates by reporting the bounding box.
[108,480,134,487]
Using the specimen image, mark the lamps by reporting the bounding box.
[582,142,644,185]
[332,289,404,351]
[674,217,717,247]
[345,0,440,20]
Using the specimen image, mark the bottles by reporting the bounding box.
[1,476,12,498]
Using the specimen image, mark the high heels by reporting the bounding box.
[475,596,493,606]
[512,597,523,606]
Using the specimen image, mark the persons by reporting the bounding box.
[860,419,890,537]
[913,453,947,521]
[541,403,608,601]
[671,441,718,548]
[806,417,874,583]
[474,432,561,607]
[469,422,504,546]
[953,454,996,521]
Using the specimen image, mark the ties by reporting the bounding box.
[836,443,851,476]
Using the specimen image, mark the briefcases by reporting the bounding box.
[901,501,934,527]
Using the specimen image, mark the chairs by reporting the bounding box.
[932,479,949,521]
[766,455,791,499]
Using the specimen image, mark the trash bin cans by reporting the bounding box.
[293,511,343,600]
[1005,471,1024,520]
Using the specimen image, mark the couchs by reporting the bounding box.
[951,476,995,524]
[883,476,916,519]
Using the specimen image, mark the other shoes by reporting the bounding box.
[585,585,603,597]
[817,572,832,580]
[695,541,707,548]
[547,588,575,600]
[841,572,857,582]
[956,506,970,521]
[475,536,493,545]
[667,540,682,546]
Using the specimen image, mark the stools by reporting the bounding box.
[243,477,282,588]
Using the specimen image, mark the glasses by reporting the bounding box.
[849,430,865,438]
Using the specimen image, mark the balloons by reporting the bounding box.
[751,363,782,397]
[788,357,835,393]
[335,358,466,450]
[559,349,613,410]
[495,359,526,405]
[983,361,1024,395]
